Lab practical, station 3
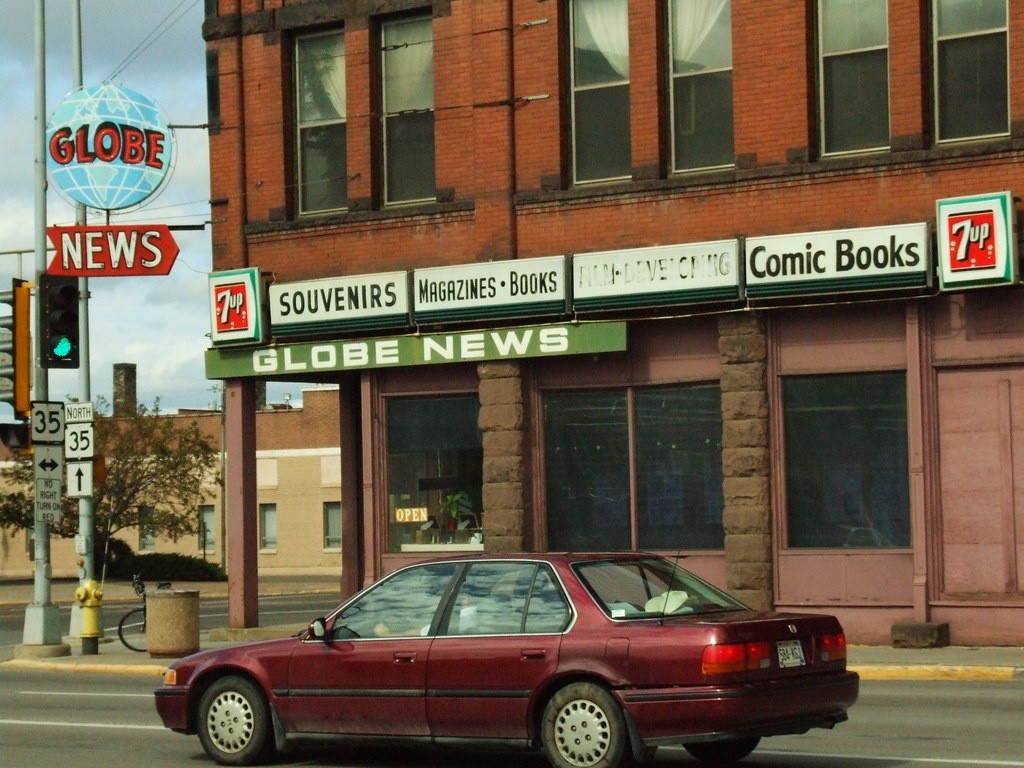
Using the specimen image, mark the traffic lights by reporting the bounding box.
[37,273,81,369]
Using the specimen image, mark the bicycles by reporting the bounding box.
[118,571,171,653]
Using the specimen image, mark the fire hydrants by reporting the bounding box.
[76,580,106,655]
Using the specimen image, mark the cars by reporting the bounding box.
[154,551,861,768]
